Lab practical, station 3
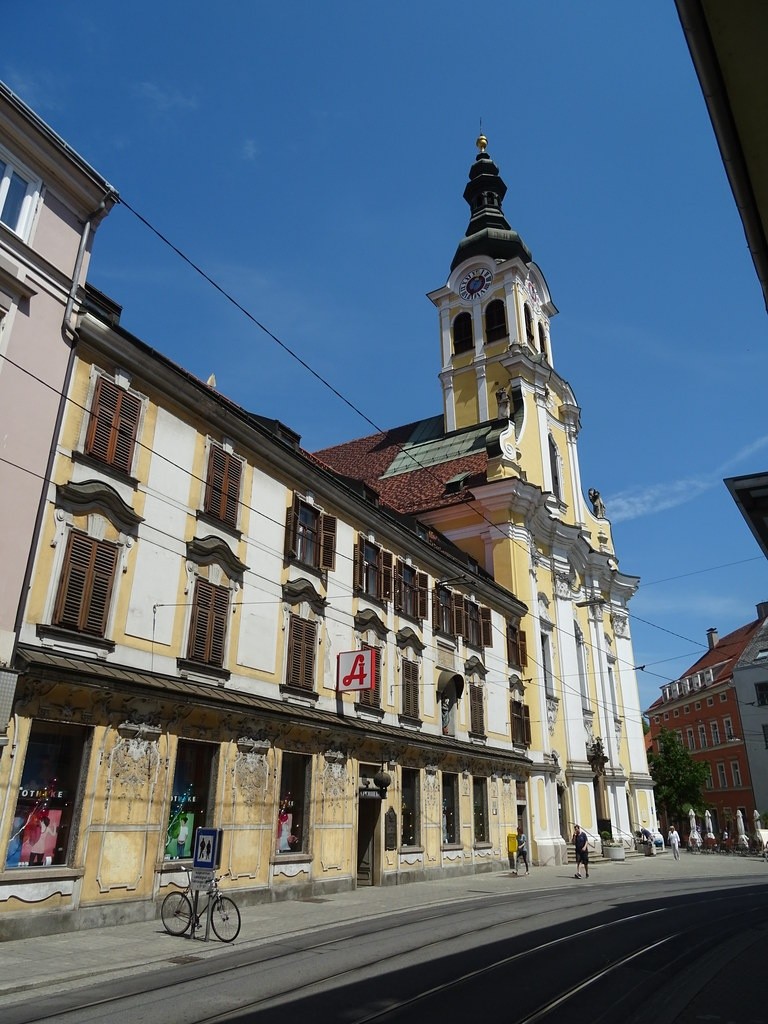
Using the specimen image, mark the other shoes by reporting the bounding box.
[674,857,680,861]
[513,870,518,875]
[585,874,590,879]
[524,871,529,874]
[575,872,581,878]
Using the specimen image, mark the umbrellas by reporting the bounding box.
[705,810,712,834]
[688,808,696,830]
[736,810,746,835]
[753,810,761,829]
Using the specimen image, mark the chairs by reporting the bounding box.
[688,837,765,857]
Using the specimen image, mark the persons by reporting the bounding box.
[572,824,590,879]
[512,825,530,875]
[668,825,681,860]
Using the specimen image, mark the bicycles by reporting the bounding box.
[691,836,762,857]
[161,873,241,943]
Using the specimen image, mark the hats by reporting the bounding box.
[670,825,674,828]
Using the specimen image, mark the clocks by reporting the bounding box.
[459,267,493,300]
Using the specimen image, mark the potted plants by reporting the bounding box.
[601,830,626,861]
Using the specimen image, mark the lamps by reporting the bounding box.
[373,751,392,799]
